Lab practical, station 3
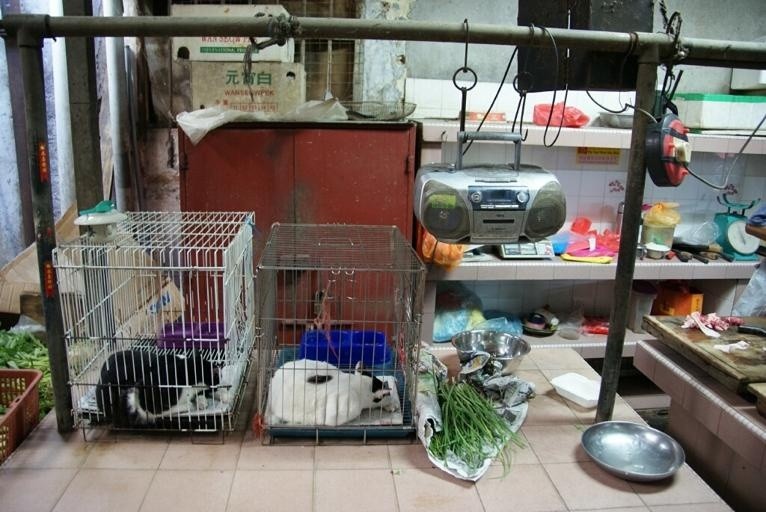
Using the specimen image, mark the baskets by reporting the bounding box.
[0,368,44,466]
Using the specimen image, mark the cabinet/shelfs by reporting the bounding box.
[409,114,766,360]
[170,116,418,348]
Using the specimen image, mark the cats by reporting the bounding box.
[267,358,399,427]
[95,348,236,426]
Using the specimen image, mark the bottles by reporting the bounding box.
[615,202,625,234]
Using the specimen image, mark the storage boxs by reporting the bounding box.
[0,201,190,347]
[168,0,307,114]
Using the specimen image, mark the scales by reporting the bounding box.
[713,194,761,261]
[496,238,556,259]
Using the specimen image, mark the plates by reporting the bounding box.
[581,421,686,483]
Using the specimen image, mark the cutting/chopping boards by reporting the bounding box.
[641,315,766,416]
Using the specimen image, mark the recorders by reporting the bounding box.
[412,131,567,245]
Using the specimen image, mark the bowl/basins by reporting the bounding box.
[450,328,532,376]
[646,243,671,259]
[599,111,634,128]
